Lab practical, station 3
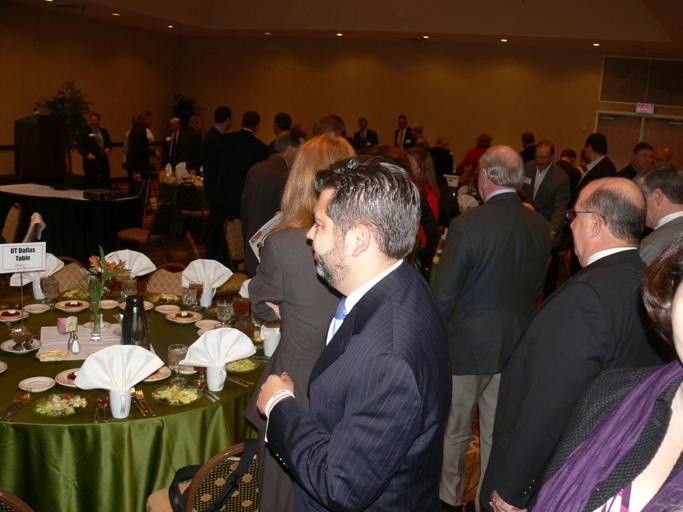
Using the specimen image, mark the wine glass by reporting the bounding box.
[233,297,251,327]
[43,277,59,310]
[234,321,255,341]
[165,170,204,182]
[189,279,204,311]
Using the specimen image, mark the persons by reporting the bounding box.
[78,107,682,303]
[249,133,355,510]
[525,231,683,511]
[432,145,549,511]
[479,176,672,511]
[255,156,451,512]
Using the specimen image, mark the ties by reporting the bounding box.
[399,129,404,147]
[170,132,177,160]
[360,130,366,143]
[93,131,104,156]
[331,297,345,337]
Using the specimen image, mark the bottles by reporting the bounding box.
[122,295,149,351]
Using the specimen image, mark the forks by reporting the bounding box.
[6,393,32,423]
[134,389,155,416]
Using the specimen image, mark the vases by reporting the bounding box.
[89,296,103,342]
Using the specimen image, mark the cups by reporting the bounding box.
[199,287,217,308]
[181,286,197,306]
[109,388,132,420]
[217,297,233,324]
[120,279,138,300]
[261,326,283,357]
[33,279,45,301]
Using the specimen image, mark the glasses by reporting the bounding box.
[566,207,606,223]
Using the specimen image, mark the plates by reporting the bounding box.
[228,361,261,375]
[33,393,85,418]
[152,386,203,409]
[1,299,232,395]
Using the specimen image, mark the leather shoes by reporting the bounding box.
[465,499,476,510]
[442,500,463,510]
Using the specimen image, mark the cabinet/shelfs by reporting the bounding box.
[14,115,66,183]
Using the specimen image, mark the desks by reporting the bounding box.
[1,182,142,251]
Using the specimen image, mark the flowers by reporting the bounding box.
[78,246,132,332]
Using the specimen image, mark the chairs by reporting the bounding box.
[222,218,248,272]
[0,489,33,512]
[144,268,183,297]
[40,263,96,301]
[146,442,260,512]
[156,182,177,205]
[174,181,204,217]
[212,272,248,303]
[117,200,176,263]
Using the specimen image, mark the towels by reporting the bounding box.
[457,184,475,195]
[181,259,233,288]
[9,252,64,286]
[166,163,172,177]
[73,343,164,392]
[457,194,479,212]
[175,162,187,185]
[103,249,157,278]
[178,327,258,367]
